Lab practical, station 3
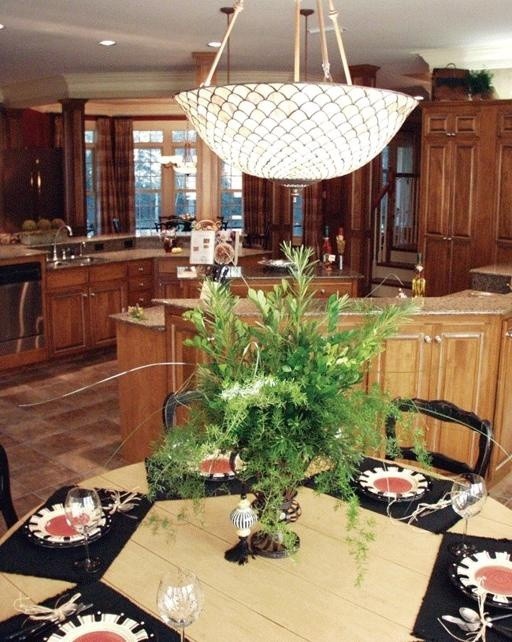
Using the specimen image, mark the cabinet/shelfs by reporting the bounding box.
[127,257,155,311]
[367,317,503,494]
[42,261,131,360]
[155,256,190,299]
[0,145,66,236]
[414,95,512,297]
[486,311,511,491]
[284,277,360,300]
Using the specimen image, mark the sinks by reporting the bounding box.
[47,260,67,269]
[66,255,109,266]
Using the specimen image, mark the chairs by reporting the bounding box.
[381,391,499,488]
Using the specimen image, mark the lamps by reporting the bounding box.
[156,118,198,178]
[169,3,423,203]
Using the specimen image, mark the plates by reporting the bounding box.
[258,258,294,274]
[187,449,249,481]
[358,465,429,502]
[452,550,512,611]
[26,500,111,551]
[43,611,151,642]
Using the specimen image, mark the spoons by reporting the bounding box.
[458,607,512,641]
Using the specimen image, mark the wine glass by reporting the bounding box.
[65,488,104,575]
[155,571,203,642]
[450,474,488,558]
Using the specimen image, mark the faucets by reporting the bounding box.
[52,224,73,260]
[80,241,87,255]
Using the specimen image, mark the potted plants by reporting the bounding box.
[143,237,426,591]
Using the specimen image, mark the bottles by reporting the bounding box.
[410,250,428,310]
[321,223,346,272]
